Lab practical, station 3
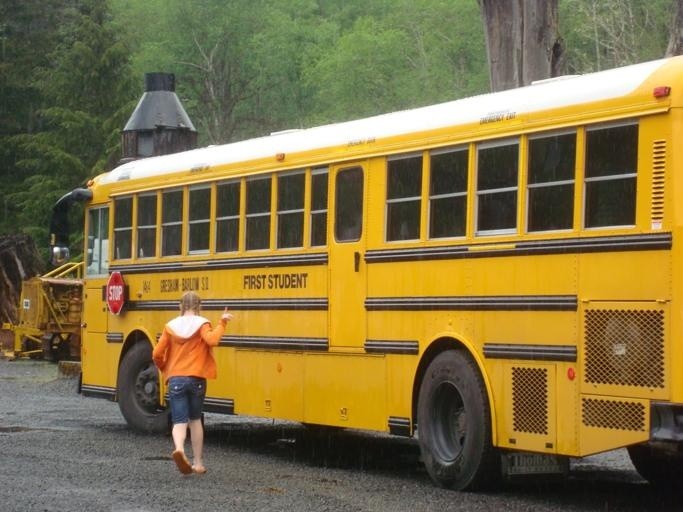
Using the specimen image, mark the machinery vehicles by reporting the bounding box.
[2,259,82,361]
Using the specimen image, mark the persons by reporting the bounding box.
[152,292,234,475]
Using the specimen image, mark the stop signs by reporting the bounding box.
[106,271,125,316]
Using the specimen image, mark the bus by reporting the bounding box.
[47,57,683,496]
